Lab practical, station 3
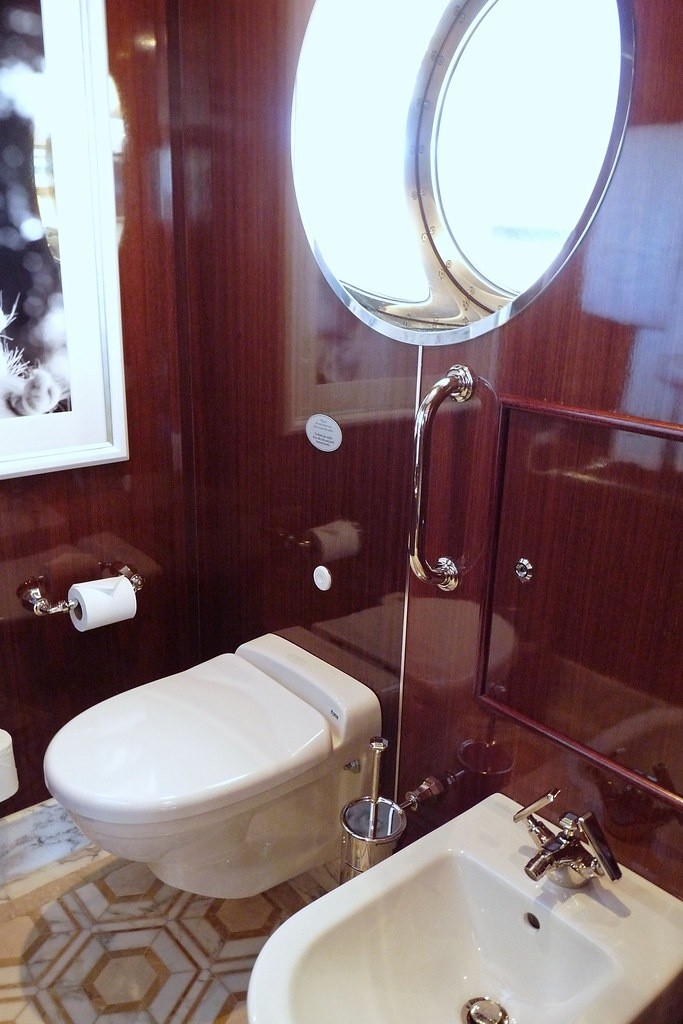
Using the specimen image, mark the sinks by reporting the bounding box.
[243,784,683,1024]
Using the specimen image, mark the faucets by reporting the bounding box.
[521,812,592,883]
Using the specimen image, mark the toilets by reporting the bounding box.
[41,629,383,900]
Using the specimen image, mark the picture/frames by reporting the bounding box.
[279,0,503,427]
[0,0,131,481]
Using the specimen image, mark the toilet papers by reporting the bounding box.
[67,572,138,632]
[308,519,359,565]
